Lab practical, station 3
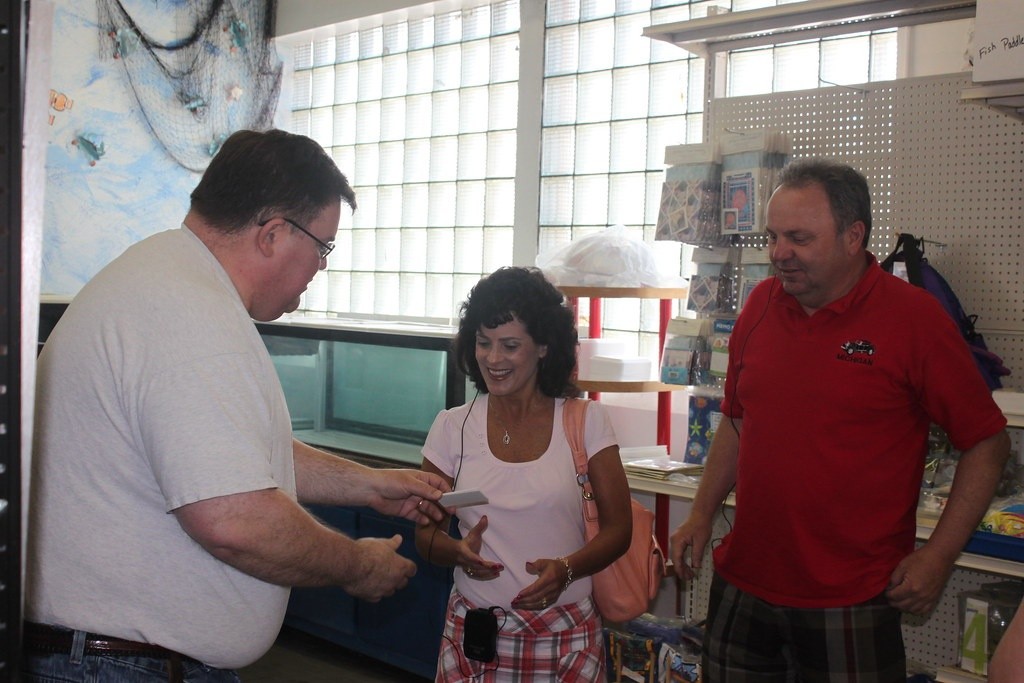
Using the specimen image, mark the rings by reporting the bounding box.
[543,598,546,608]
[468,566,474,575]
[417,500,424,508]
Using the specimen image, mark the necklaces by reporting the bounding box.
[487,400,534,443]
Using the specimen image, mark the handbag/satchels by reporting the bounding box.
[603,613,706,683]
[562,399,666,622]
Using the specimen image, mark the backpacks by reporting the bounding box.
[880,233,1012,394]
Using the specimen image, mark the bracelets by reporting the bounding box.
[555,556,573,588]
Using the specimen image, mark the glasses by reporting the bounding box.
[258,217,335,259]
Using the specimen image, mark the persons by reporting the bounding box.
[407,266,632,683]
[18,129,458,683]
[666,162,1024,683]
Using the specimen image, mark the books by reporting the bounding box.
[991,390,1024,427]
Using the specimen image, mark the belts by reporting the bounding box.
[30,629,184,657]
